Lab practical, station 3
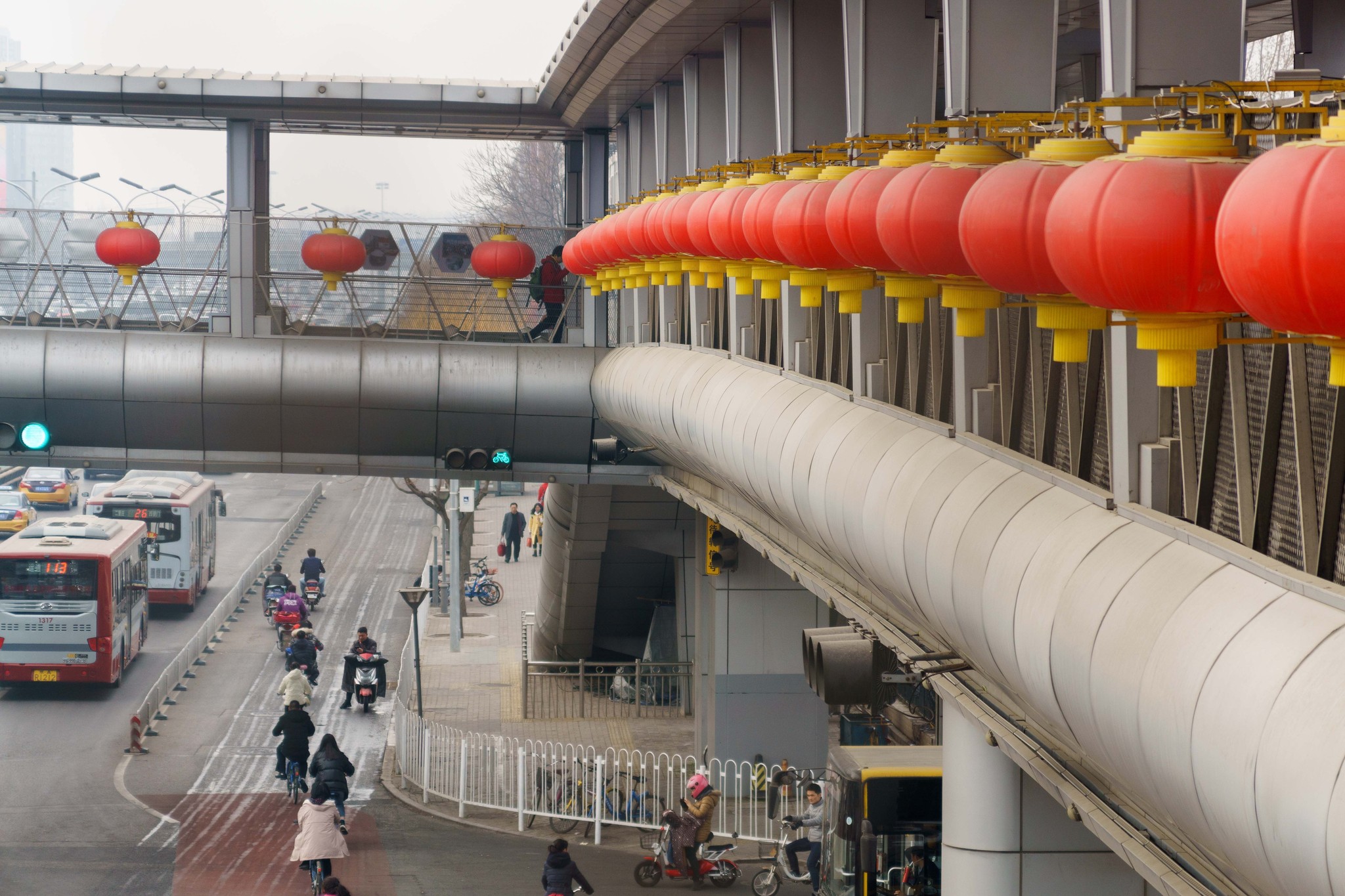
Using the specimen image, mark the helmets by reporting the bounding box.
[683,774,709,799]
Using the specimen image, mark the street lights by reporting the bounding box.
[0,168,384,327]
[395,585,435,715]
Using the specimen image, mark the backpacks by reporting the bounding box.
[528,260,556,304]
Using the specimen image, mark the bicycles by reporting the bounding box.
[292,820,338,896]
[279,733,308,804]
[526,751,667,837]
[310,774,338,829]
[571,885,587,894]
[438,556,504,606]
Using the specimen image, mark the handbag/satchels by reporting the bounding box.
[527,534,532,548]
[538,520,543,536]
[497,535,506,557]
[542,492,545,500]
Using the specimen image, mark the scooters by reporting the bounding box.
[265,573,291,629]
[287,647,315,686]
[633,809,743,887]
[344,653,378,713]
[300,558,325,612]
[750,818,820,896]
[273,611,303,656]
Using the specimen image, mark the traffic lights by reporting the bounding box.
[442,446,514,471]
[589,437,625,467]
[0,421,52,454]
[711,523,740,574]
[801,627,879,706]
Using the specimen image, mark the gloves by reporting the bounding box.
[781,815,792,825]
[791,821,803,830]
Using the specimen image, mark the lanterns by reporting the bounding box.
[470,223,534,301]
[302,218,364,293]
[559,99,1345,384]
[96,208,162,283]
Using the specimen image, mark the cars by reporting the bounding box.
[18,467,80,511]
[81,482,115,514]
[0,486,37,532]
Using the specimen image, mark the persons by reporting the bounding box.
[278,662,312,713]
[540,837,593,896]
[525,241,570,342]
[527,503,543,557]
[340,626,376,709]
[299,547,327,599]
[321,876,349,896]
[310,732,355,835]
[538,481,548,508]
[292,620,325,651]
[665,775,723,890]
[272,700,315,794]
[275,584,311,637]
[291,629,320,688]
[290,779,350,876]
[264,563,292,593]
[497,503,532,557]
[782,781,824,896]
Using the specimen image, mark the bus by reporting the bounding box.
[766,745,943,896]
[85,470,227,613]
[0,515,161,687]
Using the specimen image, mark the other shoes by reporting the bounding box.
[340,823,348,835]
[300,779,308,793]
[538,550,542,556]
[300,594,303,598]
[309,680,317,686]
[322,593,326,597]
[504,560,509,563]
[522,332,531,344]
[532,552,537,557]
[783,872,800,880]
[274,772,286,780]
[514,558,518,562]
[297,860,310,869]
[693,881,704,890]
[340,700,351,709]
